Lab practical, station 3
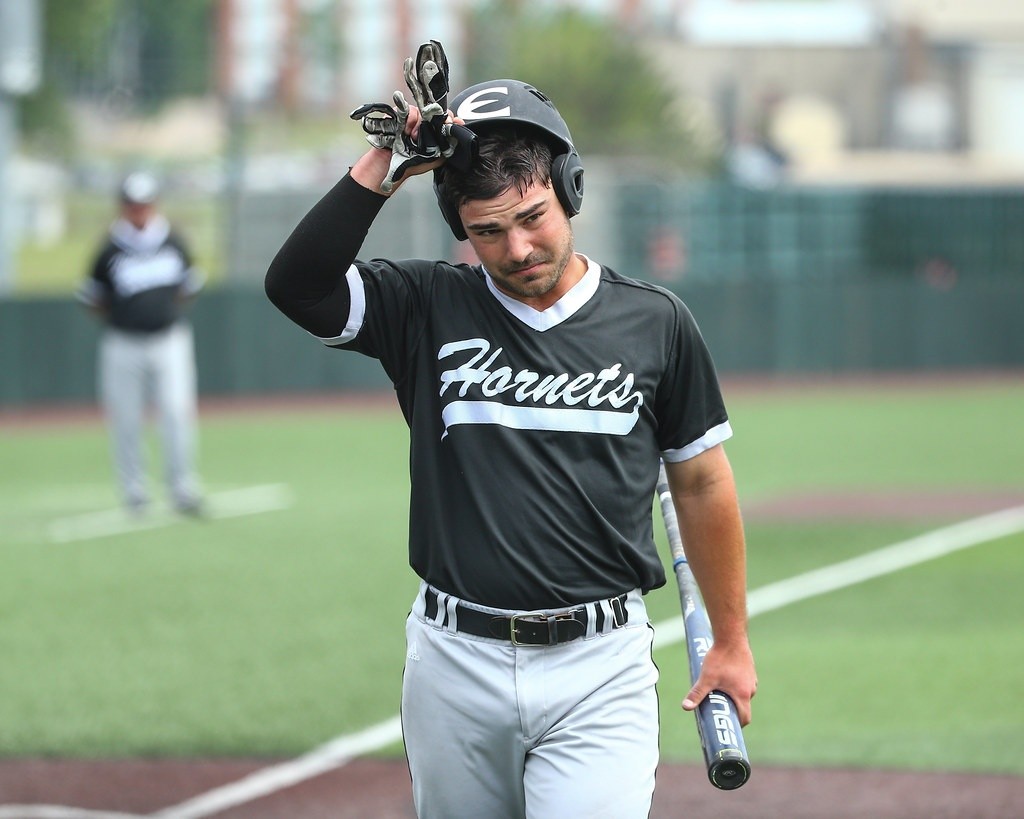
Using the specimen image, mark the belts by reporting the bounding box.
[424,585,627,647]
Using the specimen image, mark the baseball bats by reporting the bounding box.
[655,459,752,792]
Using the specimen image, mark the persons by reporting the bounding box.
[81,179,205,521]
[264,79,758,819]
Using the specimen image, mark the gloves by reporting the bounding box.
[350,40,479,192]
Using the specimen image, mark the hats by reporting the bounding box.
[121,173,157,204]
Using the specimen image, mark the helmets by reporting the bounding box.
[432,79,584,241]
